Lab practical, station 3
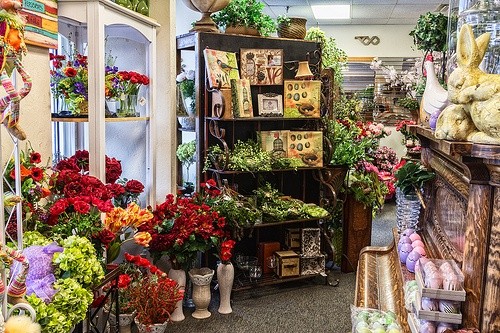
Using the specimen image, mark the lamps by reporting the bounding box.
[294,60,313,79]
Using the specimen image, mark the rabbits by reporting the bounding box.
[435,23,500,145]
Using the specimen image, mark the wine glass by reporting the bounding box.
[266,257,277,279]
[250,265,261,286]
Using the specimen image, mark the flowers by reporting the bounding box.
[0,0,453,324]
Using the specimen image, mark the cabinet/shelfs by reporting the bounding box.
[175,33,343,292]
[49,0,161,211]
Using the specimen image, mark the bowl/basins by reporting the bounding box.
[236,255,258,271]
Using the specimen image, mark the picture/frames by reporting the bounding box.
[257,93,282,115]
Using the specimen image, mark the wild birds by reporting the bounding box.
[217,59,239,77]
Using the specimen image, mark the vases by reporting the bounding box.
[134,315,168,333]
[109,310,136,333]
[394,185,422,233]
[107,99,121,112]
[166,265,188,321]
[175,86,195,128]
[188,266,214,318]
[120,95,140,117]
[217,262,234,314]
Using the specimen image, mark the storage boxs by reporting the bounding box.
[273,249,299,278]
[257,242,279,271]
